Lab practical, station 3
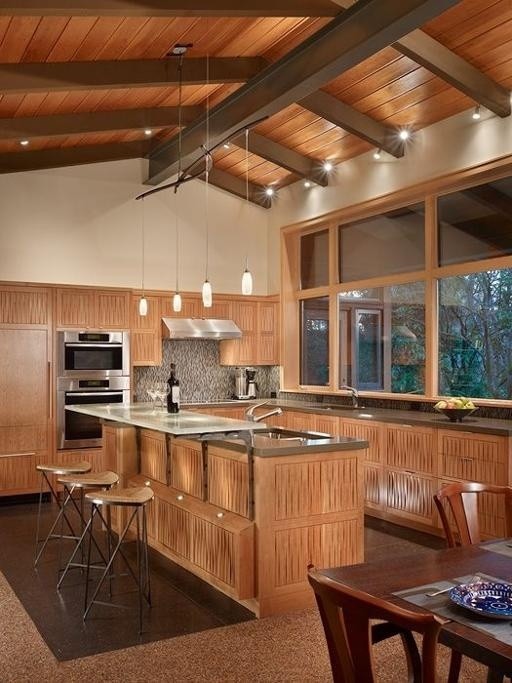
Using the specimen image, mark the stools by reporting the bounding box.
[30,458,157,640]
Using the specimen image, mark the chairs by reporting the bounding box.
[432,479,511,681]
[303,563,449,681]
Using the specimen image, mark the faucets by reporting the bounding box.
[245,400,283,421]
[339,385,357,395]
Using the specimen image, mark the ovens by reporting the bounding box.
[56,331,130,452]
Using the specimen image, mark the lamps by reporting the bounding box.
[134,43,272,316]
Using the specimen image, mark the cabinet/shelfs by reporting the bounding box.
[127,288,165,367]
[255,407,312,432]
[254,293,281,366]
[339,416,382,519]
[312,409,339,436]
[381,420,435,532]
[53,283,130,329]
[162,290,229,320]
[436,427,512,544]
[0,279,59,505]
[216,293,255,368]
[184,406,247,420]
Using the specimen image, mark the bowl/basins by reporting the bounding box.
[434,407,477,422]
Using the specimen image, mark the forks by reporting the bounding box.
[424,572,481,599]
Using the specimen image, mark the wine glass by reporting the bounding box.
[146,382,171,415]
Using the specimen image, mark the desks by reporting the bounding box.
[61,397,256,522]
[315,535,512,681]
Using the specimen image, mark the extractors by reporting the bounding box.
[162,317,242,341]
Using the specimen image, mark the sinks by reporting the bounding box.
[252,427,332,442]
[308,405,364,412]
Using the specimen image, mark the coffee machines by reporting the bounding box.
[233,367,260,399]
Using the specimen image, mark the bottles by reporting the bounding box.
[165,363,180,413]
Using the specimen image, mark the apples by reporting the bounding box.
[465,401,474,409]
[454,400,464,409]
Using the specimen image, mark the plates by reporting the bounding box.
[447,579,511,621]
